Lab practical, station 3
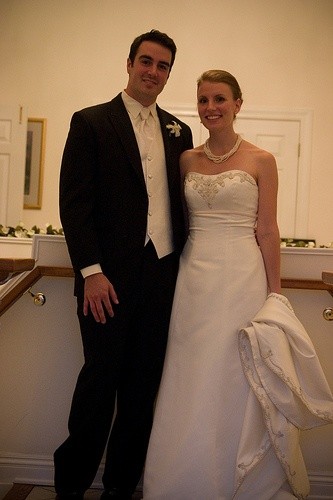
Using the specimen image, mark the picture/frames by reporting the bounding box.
[23,118,47,211]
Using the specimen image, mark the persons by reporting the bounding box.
[142,70,333,500]
[54,29,193,500]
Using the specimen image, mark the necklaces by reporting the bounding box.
[204,133,242,163]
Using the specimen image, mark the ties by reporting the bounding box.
[139,106,155,136]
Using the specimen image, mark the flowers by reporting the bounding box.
[0,222,64,238]
[167,121,182,138]
[280,239,332,248]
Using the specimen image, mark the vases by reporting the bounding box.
[279,248,333,280]
[31,234,72,267]
[0,236,34,258]
